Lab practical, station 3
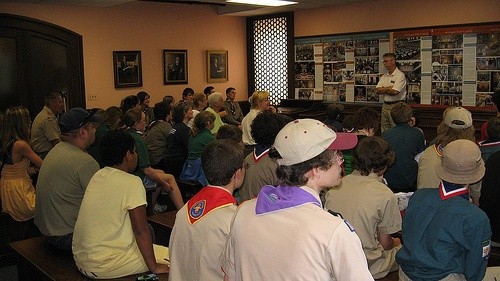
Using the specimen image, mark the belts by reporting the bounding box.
[384,101,404,105]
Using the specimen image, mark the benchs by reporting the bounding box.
[9,236,169,281]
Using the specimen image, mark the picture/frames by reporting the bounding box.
[206,50,230,84]
[163,49,188,85]
[113,50,142,88]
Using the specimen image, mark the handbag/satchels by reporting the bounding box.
[179,158,206,186]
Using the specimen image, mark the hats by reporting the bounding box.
[435,139,486,185]
[442,105,473,129]
[273,117,358,167]
[57,108,98,133]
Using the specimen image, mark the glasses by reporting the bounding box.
[325,152,344,165]
[382,59,393,63]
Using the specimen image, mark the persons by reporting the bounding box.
[375,53,407,130]
[66,129,170,281]
[169,138,245,281]
[0,85,496,213]
[34,108,110,244]
[0,113,47,224]
[396,136,490,281]
[219,114,379,281]
[324,133,407,281]
